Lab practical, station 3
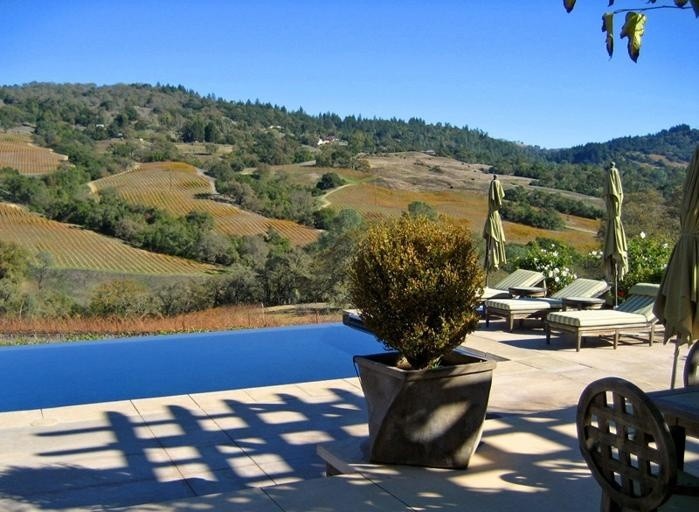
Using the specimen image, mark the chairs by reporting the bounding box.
[474,268,662,351]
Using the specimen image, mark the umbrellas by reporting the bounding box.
[483,174,508,285]
[600,161,630,306]
[650,145,699,390]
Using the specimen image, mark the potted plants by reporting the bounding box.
[344,207,496,471]
